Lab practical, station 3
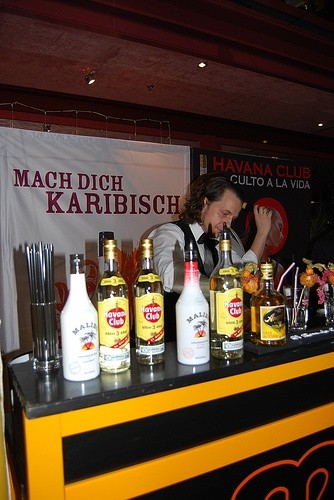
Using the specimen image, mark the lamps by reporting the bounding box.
[84,68,98,84]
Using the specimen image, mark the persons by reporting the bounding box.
[147,171,274,341]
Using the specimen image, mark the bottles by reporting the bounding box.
[60,253,100,382]
[209,222,244,361]
[132,238,166,366]
[175,240,210,366]
[91,229,114,311]
[250,261,287,346]
[96,239,131,375]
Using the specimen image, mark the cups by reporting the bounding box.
[281,286,309,334]
[30,300,60,377]
[324,283,334,328]
